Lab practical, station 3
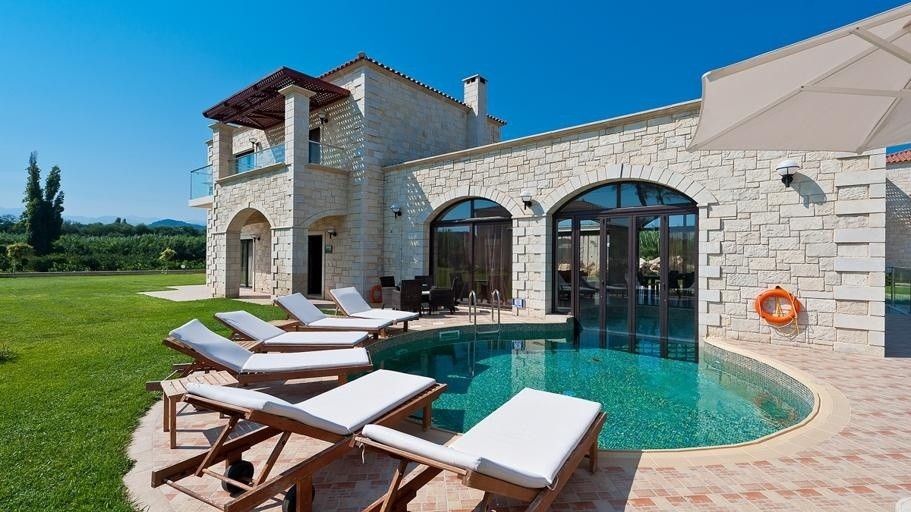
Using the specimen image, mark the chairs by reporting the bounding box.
[145,318,372,396]
[379,276,464,315]
[212,311,374,350]
[275,293,395,341]
[355,387,610,510]
[145,275,609,512]
[558,270,695,301]
[151,368,447,508]
[328,286,419,337]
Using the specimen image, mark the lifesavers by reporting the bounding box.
[370,284,381,303]
[755,289,801,323]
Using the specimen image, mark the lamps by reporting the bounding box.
[318,110,328,126]
[327,225,338,240]
[776,162,799,187]
[520,190,532,210]
[390,201,402,220]
[249,132,260,149]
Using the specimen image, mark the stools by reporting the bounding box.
[161,371,240,449]
[266,319,301,332]
[229,339,265,354]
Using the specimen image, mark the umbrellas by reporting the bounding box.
[681,2,911,156]
